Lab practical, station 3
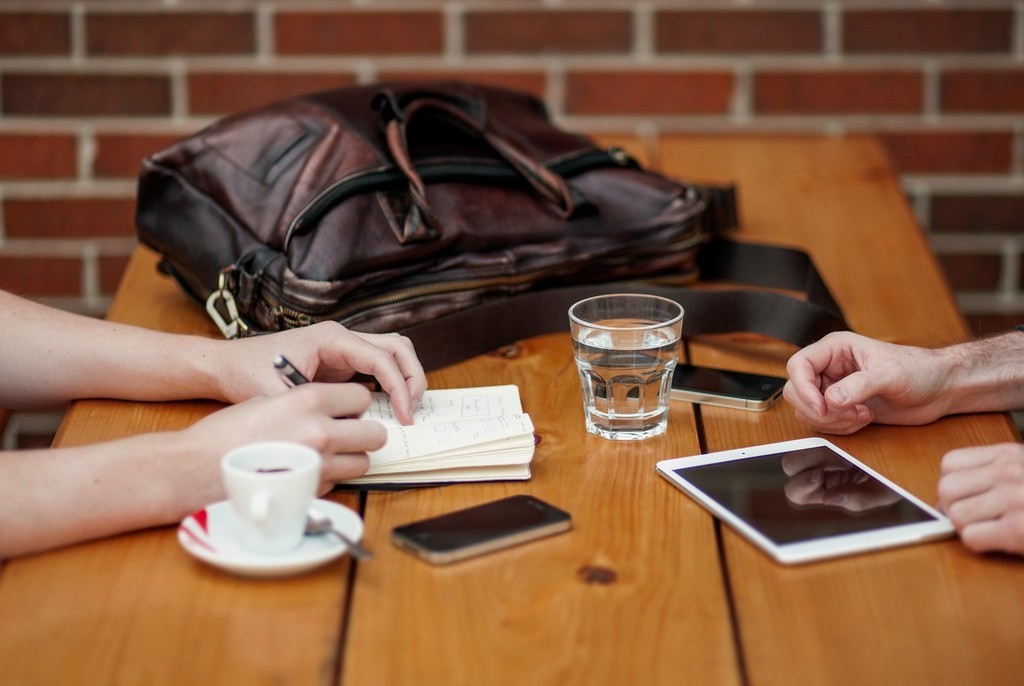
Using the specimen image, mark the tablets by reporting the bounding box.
[655,438,955,566]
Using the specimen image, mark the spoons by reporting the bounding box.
[305,510,374,561]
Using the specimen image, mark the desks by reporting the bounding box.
[1,128,1023,686]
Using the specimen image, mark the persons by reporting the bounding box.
[0,287,428,564]
[783,328,1023,557]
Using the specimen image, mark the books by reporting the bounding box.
[329,385,536,483]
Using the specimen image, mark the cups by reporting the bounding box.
[220,441,323,555]
[569,294,684,441]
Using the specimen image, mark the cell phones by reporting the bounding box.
[669,362,788,412]
[392,495,572,566]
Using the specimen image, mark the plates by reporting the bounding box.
[178,500,363,578]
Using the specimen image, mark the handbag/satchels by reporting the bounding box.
[136,78,856,394]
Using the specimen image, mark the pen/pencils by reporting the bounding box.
[272,352,310,387]
